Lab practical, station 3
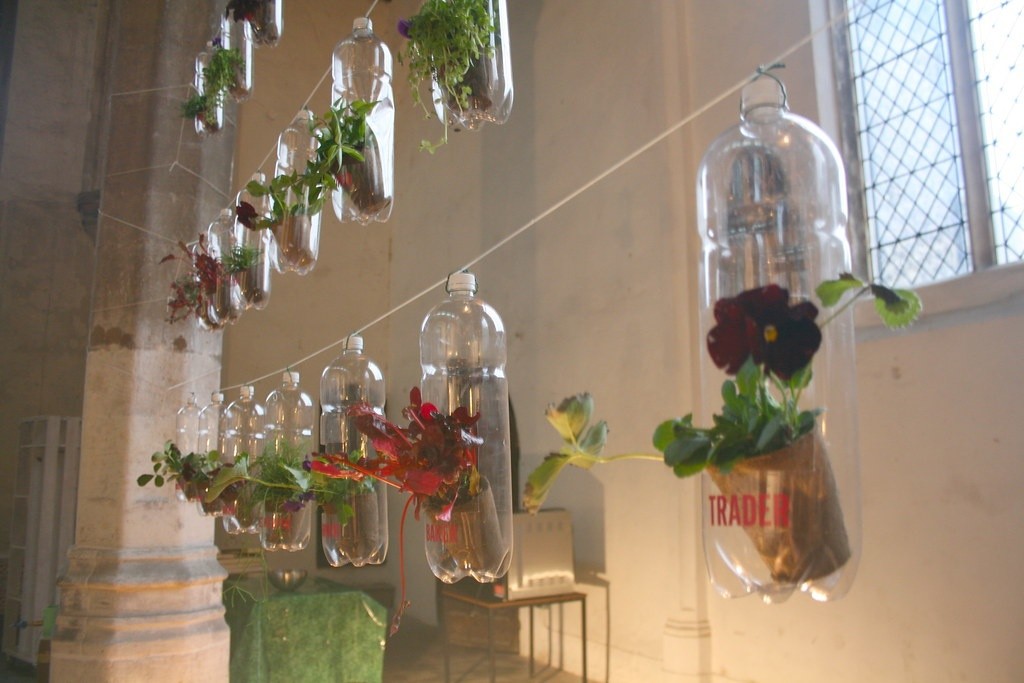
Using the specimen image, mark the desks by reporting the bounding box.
[224,570,387,683]
[435,582,587,683]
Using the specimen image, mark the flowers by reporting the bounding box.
[136,437,388,526]
[155,229,257,326]
[307,384,480,638]
[235,98,391,261]
[201,36,245,91]
[521,269,922,516]
[223,0,266,24]
[398,0,496,156]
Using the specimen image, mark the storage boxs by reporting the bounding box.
[471,508,576,601]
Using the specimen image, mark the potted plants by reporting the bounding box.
[181,94,227,135]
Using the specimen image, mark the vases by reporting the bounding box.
[219,64,250,94]
[335,149,391,215]
[321,493,386,566]
[249,8,278,44]
[265,216,313,270]
[408,481,509,583]
[692,413,856,603]
[438,55,492,113]
[235,267,265,304]
[261,502,305,556]
